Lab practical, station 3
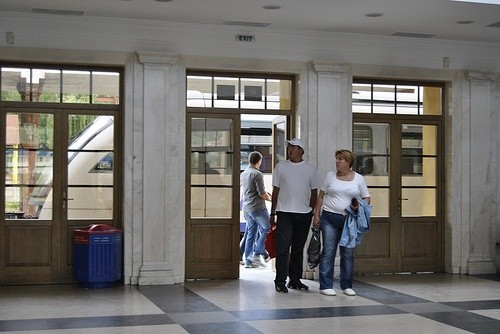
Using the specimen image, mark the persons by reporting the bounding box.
[269,138,322,293]
[239,151,272,268]
[313,150,371,296]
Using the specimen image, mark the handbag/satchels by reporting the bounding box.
[266,223,277,259]
[307,224,321,269]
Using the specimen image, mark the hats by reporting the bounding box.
[287,138,305,152]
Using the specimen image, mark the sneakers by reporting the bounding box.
[320,289,336,296]
[252,252,268,267]
[343,288,356,295]
[244,262,260,268]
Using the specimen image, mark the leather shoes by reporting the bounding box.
[275,284,288,293]
[287,277,308,290]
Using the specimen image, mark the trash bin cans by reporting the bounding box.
[72,223,123,288]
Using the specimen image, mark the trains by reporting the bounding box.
[20,86,423,237]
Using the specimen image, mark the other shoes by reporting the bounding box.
[240,259,246,266]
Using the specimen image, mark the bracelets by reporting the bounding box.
[270,213,276,217]
